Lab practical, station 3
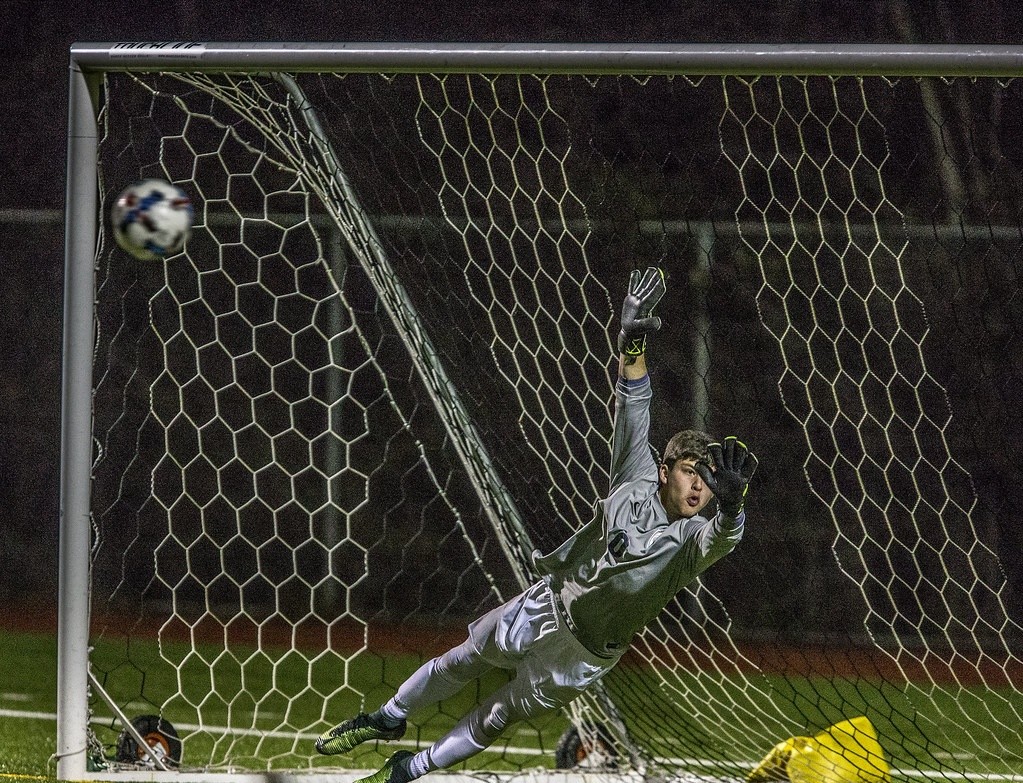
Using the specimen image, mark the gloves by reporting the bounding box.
[618,267,667,355]
[694,435,759,518]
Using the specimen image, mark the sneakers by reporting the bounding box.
[315,711,407,755]
[354,750,417,783]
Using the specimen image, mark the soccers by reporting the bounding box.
[111,179,196,263]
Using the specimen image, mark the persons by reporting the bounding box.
[315,265,760,783]
